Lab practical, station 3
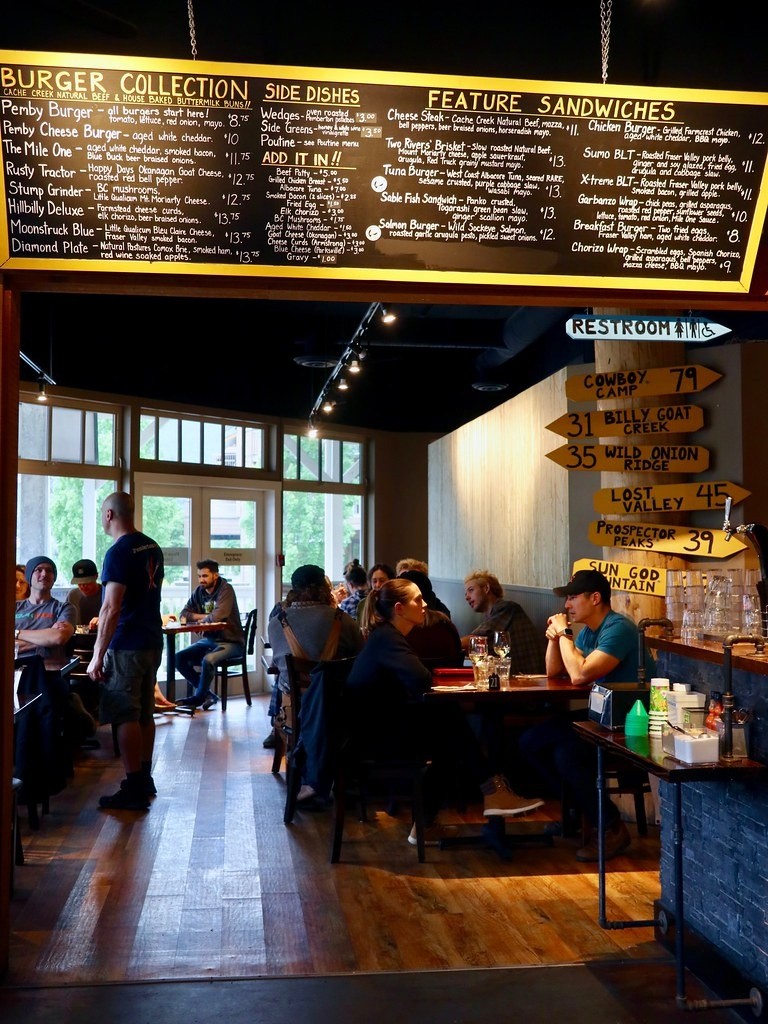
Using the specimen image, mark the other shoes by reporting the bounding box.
[68,692,97,737]
[154,697,177,709]
[203,692,219,709]
[175,696,199,707]
[99,780,150,809]
[295,792,324,811]
[263,728,275,747]
[142,777,158,795]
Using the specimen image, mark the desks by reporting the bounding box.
[159,620,230,714]
[14,625,101,864]
[410,676,589,859]
[574,717,762,1013]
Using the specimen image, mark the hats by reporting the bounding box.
[397,571,432,596]
[73,559,97,584]
[554,571,610,596]
[26,556,57,587]
[292,565,325,592]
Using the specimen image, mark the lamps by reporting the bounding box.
[307,303,396,436]
[38,379,47,401]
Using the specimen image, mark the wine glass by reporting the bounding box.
[468,636,489,686]
[494,631,511,658]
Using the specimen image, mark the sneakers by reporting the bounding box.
[576,819,631,862]
[480,774,545,817]
[408,820,461,845]
[544,820,568,836]
[565,808,591,847]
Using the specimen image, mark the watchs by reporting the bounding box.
[556,629,573,637]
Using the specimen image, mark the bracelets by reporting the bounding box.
[15,630,19,638]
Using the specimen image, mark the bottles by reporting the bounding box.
[704,690,716,729]
[712,693,723,731]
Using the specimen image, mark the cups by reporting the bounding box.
[488,656,494,676]
[14,642,19,660]
[648,677,670,735]
[180,613,186,625]
[493,658,510,680]
[161,615,169,626]
[476,662,494,688]
[76,625,90,634]
[666,567,762,640]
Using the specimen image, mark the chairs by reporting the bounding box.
[186,610,256,711]
[283,654,425,867]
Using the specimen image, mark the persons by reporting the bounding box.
[66,559,102,630]
[408,610,463,671]
[460,571,546,675]
[88,492,165,813]
[174,560,245,710]
[544,569,658,862]
[15,556,96,732]
[155,615,178,706]
[269,565,362,695]
[340,559,369,620]
[263,602,284,747]
[396,560,450,619]
[357,563,394,625]
[345,579,544,845]
